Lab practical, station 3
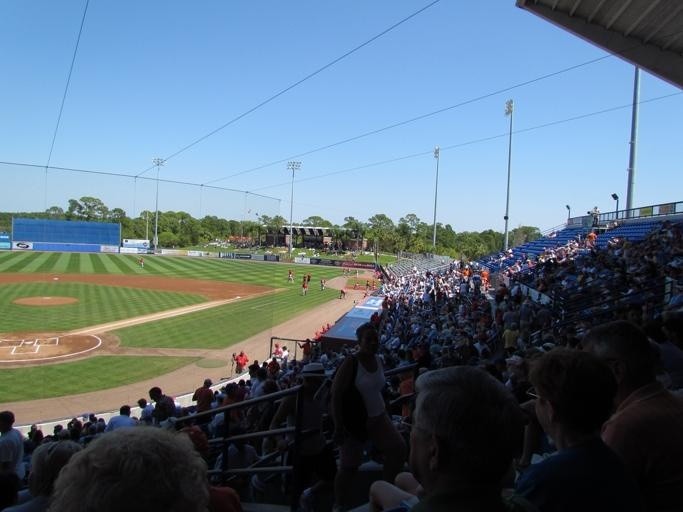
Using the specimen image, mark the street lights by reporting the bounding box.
[611,192,618,219]
[151,157,168,250]
[500,98,517,253]
[431,144,440,250]
[285,160,304,256]
[565,203,571,219]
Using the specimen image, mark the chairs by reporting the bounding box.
[387,254,474,299]
[474,215,669,297]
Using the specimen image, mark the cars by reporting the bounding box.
[202,238,261,249]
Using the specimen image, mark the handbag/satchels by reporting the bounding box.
[313,378,368,426]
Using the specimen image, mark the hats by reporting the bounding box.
[295,363,334,377]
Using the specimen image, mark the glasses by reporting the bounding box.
[402,416,427,437]
[526,387,543,400]
